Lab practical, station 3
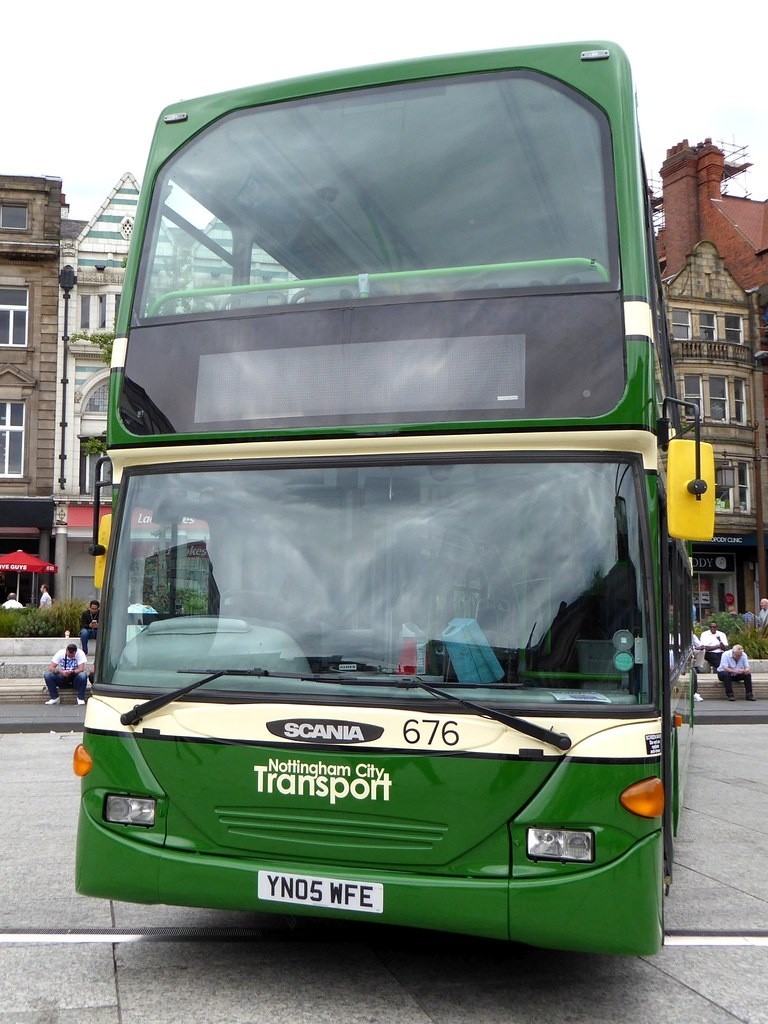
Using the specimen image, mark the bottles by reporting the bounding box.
[398,624,417,674]
[412,625,428,676]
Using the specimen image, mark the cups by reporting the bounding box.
[65,630,70,639]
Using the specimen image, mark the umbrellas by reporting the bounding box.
[0,550,58,602]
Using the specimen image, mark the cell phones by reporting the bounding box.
[92,620,97,624]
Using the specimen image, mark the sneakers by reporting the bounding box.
[77,697,85,705]
[694,693,703,702]
[44,697,60,705]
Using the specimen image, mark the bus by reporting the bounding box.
[73,40,717,958]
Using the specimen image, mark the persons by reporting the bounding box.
[80,600,100,655]
[717,645,756,701]
[729,606,738,620]
[2,593,23,609]
[701,621,728,668]
[44,644,87,705]
[760,599,768,627]
[692,634,706,701]
[39,584,52,609]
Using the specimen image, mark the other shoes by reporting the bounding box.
[729,695,735,702]
[84,650,89,656]
[746,695,756,702]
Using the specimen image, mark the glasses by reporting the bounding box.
[710,626,716,628]
